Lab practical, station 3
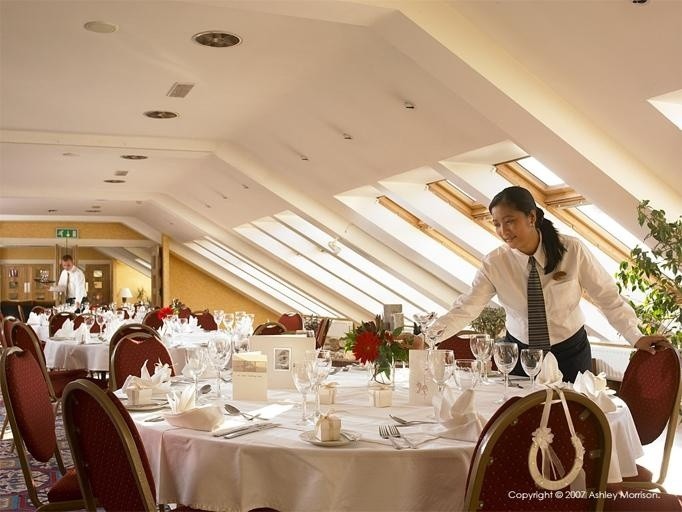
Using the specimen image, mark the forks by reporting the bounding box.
[376,424,417,454]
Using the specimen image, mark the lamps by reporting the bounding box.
[118,287,133,304]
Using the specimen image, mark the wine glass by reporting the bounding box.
[183,311,545,429]
[43,301,254,342]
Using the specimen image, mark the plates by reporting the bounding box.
[297,426,362,447]
[124,404,162,411]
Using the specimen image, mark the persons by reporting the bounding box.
[392,186,669,383]
[40,254,88,310]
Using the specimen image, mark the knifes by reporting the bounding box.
[210,423,281,441]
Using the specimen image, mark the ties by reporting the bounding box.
[526,256,550,351]
[66,273,71,298]
